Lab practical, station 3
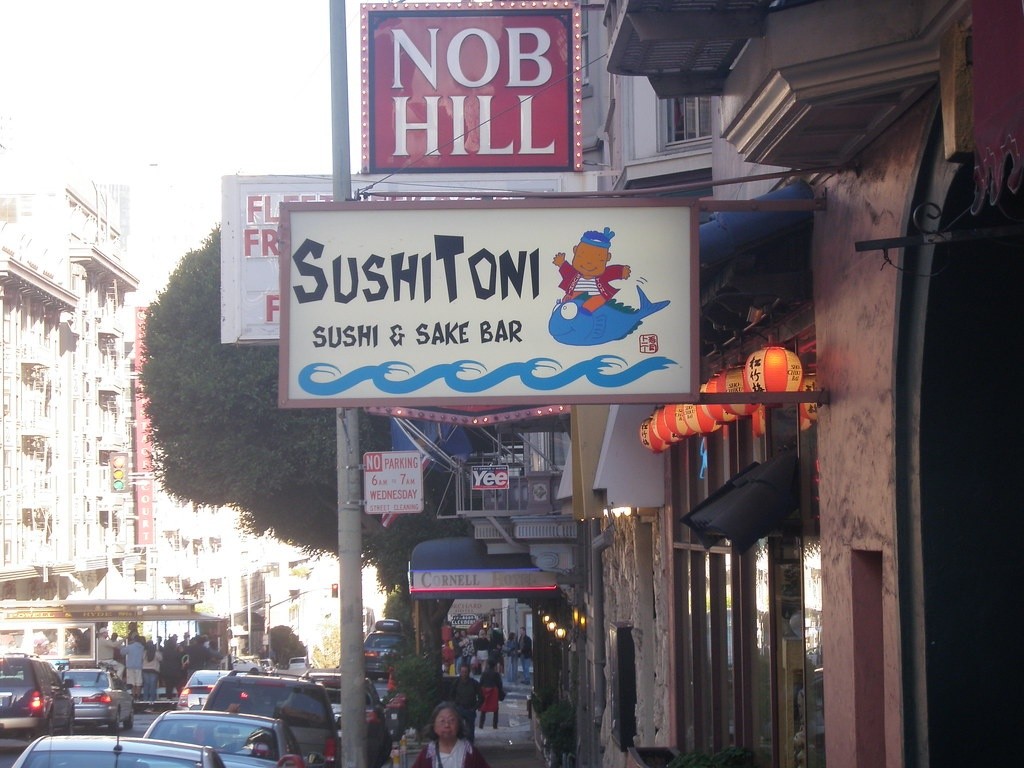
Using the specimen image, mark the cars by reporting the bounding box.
[173,656,407,768]
[138,709,327,768]
[10,734,227,768]
[58,665,136,733]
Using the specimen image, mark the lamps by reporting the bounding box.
[536,603,587,650]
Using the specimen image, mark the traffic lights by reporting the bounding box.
[332,583,339,599]
[110,452,129,493]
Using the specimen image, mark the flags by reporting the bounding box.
[381,417,472,531]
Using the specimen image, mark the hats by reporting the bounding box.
[99,627,108,633]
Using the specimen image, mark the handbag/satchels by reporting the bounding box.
[502,645,512,653]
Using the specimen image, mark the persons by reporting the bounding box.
[410,701,489,768]
[98,621,224,701]
[479,658,502,729]
[447,665,483,741]
[442,621,532,684]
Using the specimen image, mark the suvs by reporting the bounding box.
[0,652,75,741]
[190,667,341,768]
[362,618,408,682]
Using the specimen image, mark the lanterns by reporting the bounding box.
[640,346,803,454]
[799,375,817,424]
